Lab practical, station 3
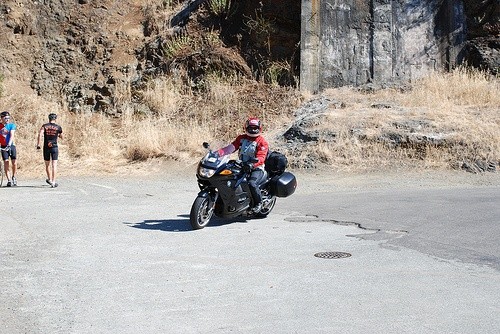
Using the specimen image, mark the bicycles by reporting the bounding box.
[0,147,13,188]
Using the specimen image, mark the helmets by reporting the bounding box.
[243,118,262,137]
[0,111,9,117]
[48,113,57,119]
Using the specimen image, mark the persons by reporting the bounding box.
[0,111,17,187]
[215,117,268,212]
[37,113,63,187]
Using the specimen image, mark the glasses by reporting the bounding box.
[249,127,257,130]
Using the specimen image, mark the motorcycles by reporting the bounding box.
[188,142,298,229]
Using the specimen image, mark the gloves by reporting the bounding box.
[244,164,254,172]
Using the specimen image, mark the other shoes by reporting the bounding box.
[7,182,11,186]
[46,179,56,188]
[253,201,263,213]
[12,176,16,185]
